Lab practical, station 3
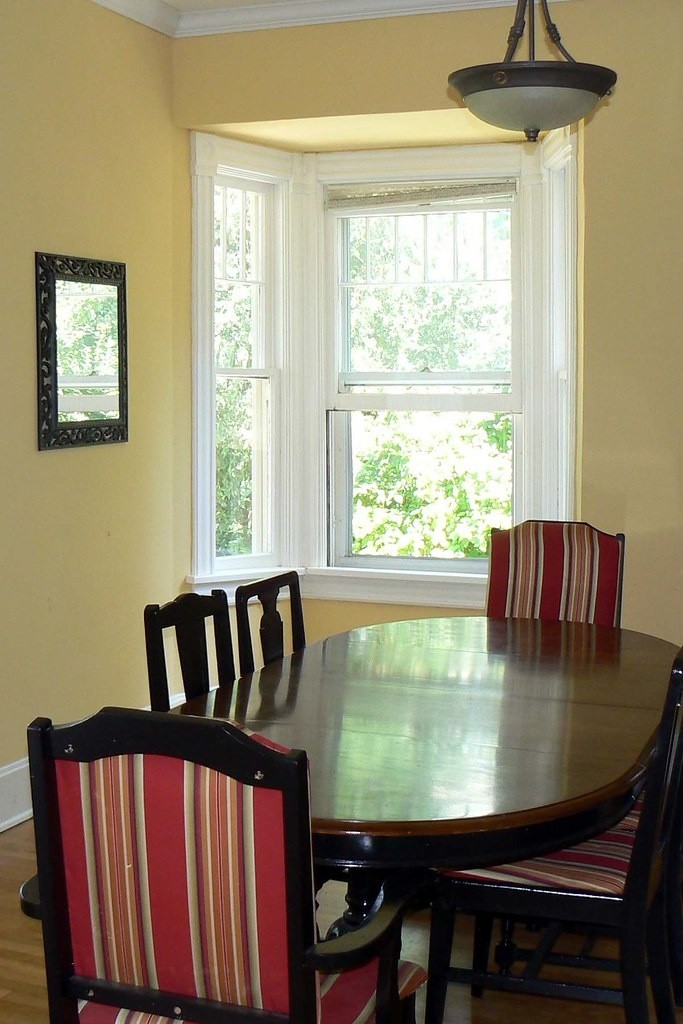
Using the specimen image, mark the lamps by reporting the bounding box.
[448,0,617,145]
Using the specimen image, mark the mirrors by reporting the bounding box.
[34,251,130,452]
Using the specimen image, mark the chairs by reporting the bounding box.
[484,519,626,626]
[19,706,429,1024]
[143,589,236,712]
[235,570,306,677]
[426,646,683,1024]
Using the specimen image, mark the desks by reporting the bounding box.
[173,615,683,1024]
